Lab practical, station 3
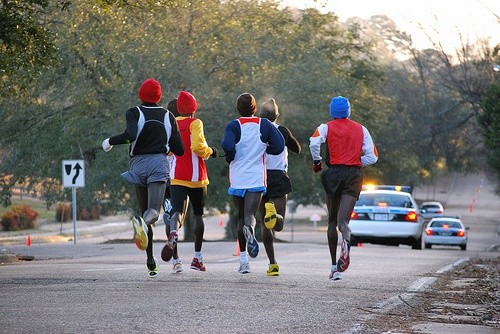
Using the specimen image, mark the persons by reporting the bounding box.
[221,93,285,273]
[162,98,190,272]
[101,78,185,276]
[309,96,378,280]
[259,98,302,275]
[160,92,218,271]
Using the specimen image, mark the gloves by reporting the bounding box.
[210,147,218,158]
[102,138,113,153]
[312,161,323,173]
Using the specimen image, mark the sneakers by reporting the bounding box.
[238,263,251,272]
[337,239,351,272]
[161,234,178,262]
[190,258,205,271]
[329,271,342,280]
[132,216,148,250]
[146,262,158,277]
[264,201,277,230]
[266,264,279,276]
[172,261,183,272]
[242,224,259,258]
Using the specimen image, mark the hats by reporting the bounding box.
[259,98,279,119]
[139,78,162,103]
[177,91,198,114]
[167,99,180,117]
[236,92,257,116]
[330,96,351,118]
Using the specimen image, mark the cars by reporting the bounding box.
[421,202,444,220]
[425,216,470,251]
[347,185,425,250]
